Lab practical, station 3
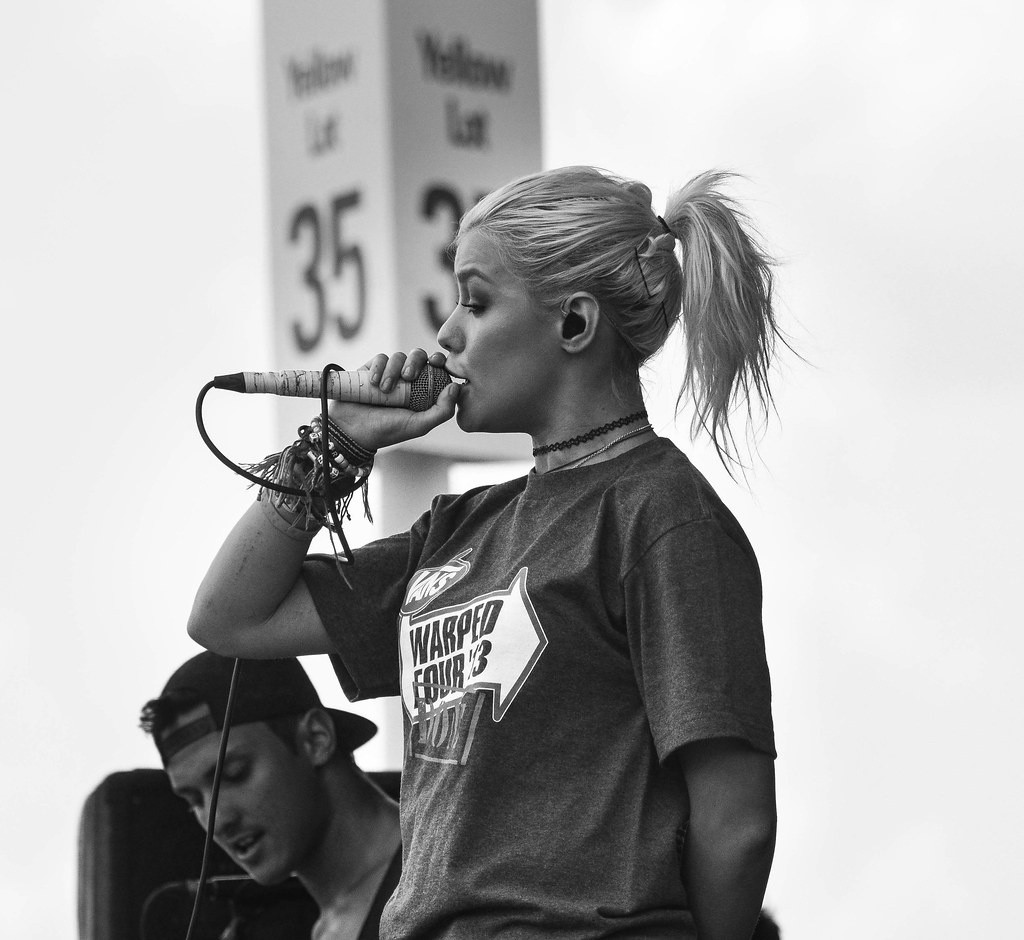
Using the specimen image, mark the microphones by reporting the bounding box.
[213,362,453,413]
[188,872,305,899]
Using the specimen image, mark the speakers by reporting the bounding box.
[73,767,403,940]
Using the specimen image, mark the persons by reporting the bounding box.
[140,648,403,940]
[190,167,790,939]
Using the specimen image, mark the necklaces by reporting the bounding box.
[531,410,655,475]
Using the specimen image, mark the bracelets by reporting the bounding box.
[236,410,374,543]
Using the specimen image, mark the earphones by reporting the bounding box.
[562,311,586,341]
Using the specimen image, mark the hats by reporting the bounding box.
[152,648,379,768]
